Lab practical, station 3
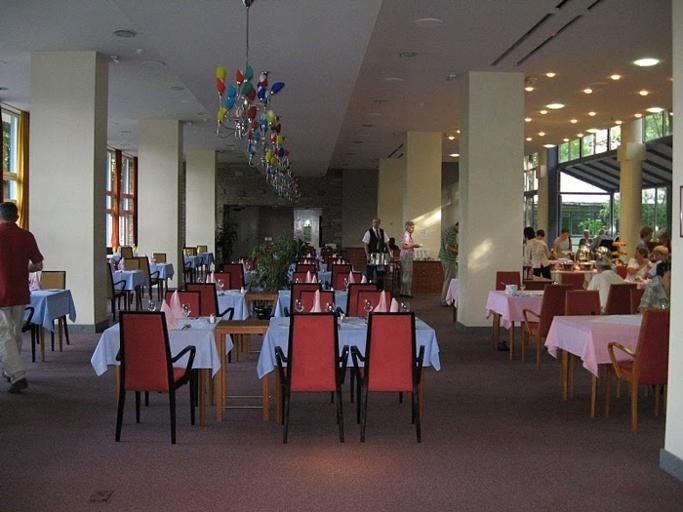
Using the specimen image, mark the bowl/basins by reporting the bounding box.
[560,259,597,272]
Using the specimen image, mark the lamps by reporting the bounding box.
[215,0,304,207]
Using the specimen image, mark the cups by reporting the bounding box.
[415,250,428,261]
[505,285,518,294]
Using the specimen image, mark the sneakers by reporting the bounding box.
[2,371,27,392]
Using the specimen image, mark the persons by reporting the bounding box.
[521,225,670,314]
[388,236,399,258]
[398,220,423,299]
[437,221,459,307]
[362,217,393,291]
[0,202,43,394]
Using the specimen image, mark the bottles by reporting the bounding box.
[370,246,390,265]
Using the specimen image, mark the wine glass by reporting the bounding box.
[294,296,411,328]
[196,246,366,293]
[106,244,140,270]
[519,284,526,295]
[144,299,216,324]
[658,298,668,310]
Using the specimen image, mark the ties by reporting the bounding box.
[376,229,380,239]
